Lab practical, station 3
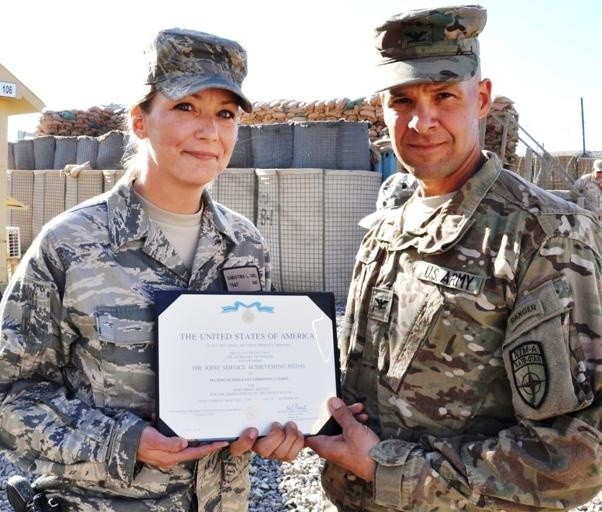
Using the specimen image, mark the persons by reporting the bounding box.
[570,156,602,222]
[0,25,307,512]
[305,2,602,512]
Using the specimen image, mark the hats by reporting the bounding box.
[592,160,602,174]
[141,28,255,114]
[369,3,488,96]
